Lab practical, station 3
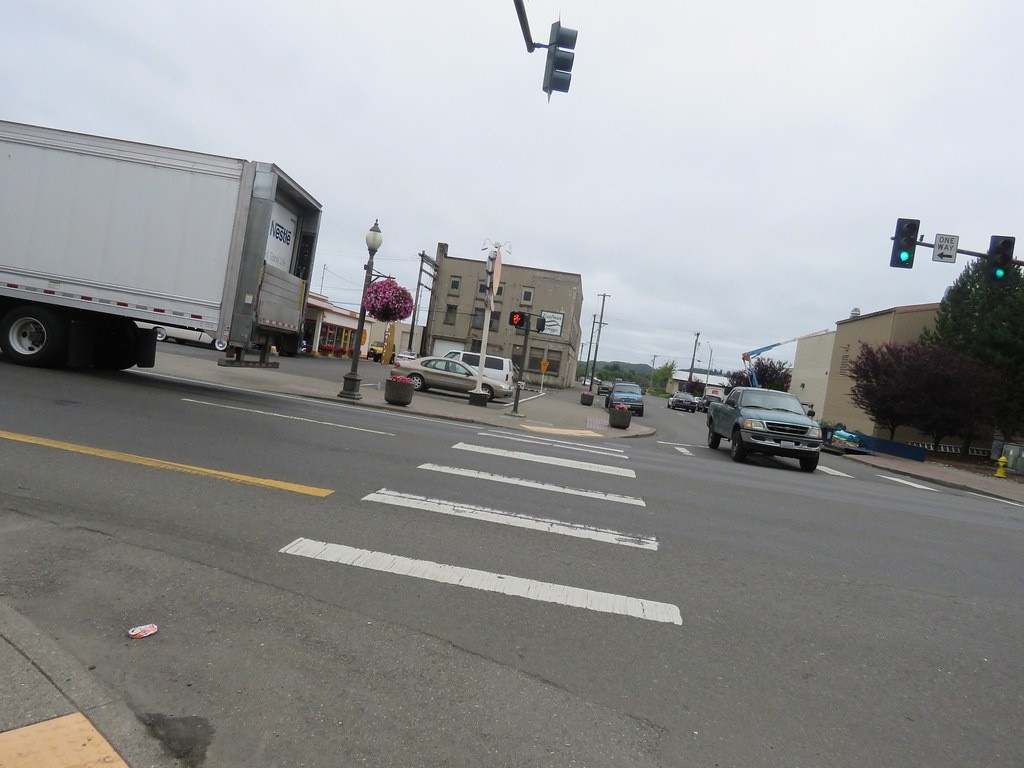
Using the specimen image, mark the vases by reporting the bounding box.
[322,349,329,356]
[334,351,343,358]
[609,408,635,429]
[384,380,415,406]
[580,394,594,406]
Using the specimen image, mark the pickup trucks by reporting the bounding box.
[706,386,824,475]
[697,395,724,414]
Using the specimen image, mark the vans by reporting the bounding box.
[432,350,514,388]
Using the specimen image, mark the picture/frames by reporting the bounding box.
[537,310,564,336]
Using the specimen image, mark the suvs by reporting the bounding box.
[366,341,396,365]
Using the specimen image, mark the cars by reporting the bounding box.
[390,356,514,403]
[668,392,698,413]
[395,351,422,363]
[580,375,646,417]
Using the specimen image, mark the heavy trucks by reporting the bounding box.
[0,119,323,374]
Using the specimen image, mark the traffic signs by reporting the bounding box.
[933,234,959,263]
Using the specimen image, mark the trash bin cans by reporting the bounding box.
[821,426,834,444]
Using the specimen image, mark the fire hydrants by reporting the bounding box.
[994,456,1008,477]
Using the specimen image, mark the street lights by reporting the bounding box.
[468,239,513,407]
[337,219,383,400]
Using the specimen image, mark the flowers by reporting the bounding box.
[322,343,336,352]
[581,389,592,396]
[387,375,414,384]
[338,345,347,354]
[362,280,412,322]
[615,404,631,411]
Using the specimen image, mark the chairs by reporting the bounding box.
[449,364,456,372]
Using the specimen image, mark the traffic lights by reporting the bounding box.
[890,218,920,269]
[985,236,1015,290]
[508,311,525,327]
[542,21,578,103]
[537,317,546,331]
